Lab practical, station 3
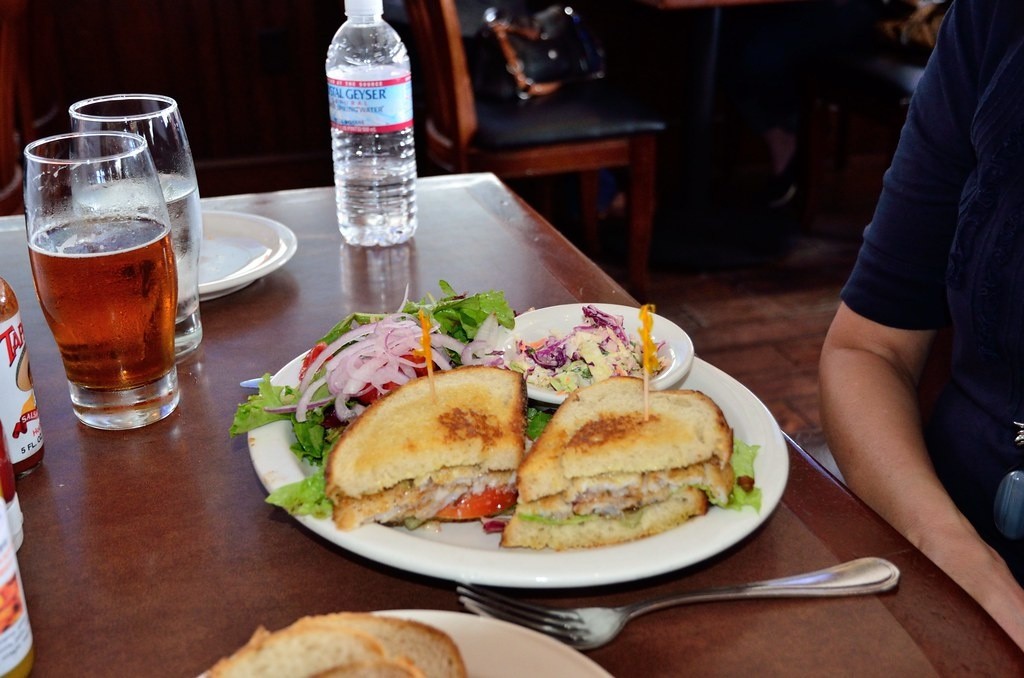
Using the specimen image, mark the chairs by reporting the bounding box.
[399,0,666,311]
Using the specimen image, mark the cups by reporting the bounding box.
[23,130,183,431]
[69,93,204,365]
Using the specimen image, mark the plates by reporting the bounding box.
[190,610,616,678]
[248,329,790,590]
[493,304,697,408]
[192,206,298,302]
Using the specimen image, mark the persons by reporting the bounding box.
[817,0,1024,651]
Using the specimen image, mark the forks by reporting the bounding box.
[457,557,902,650]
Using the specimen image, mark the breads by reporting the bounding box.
[200,612,470,678]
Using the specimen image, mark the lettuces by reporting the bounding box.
[230,281,518,517]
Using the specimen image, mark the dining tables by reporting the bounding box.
[0,170,1024,678]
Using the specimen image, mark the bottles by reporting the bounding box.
[0,497,38,677]
[0,426,25,552]
[0,275,48,475]
[323,0,417,247]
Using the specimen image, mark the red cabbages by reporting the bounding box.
[503,305,665,381]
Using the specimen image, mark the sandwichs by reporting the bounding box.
[492,372,761,553]
[326,364,532,531]
